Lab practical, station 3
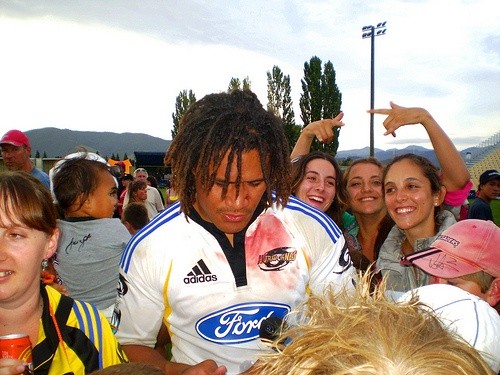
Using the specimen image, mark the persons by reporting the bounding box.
[290,101,474,286]
[395,284,499,374]
[466,170,500,225]
[0,170,128,375]
[399,219,500,313]
[349,253,377,298]
[376,153,459,292]
[49,145,133,324]
[0,129,51,193]
[112,88,372,375]
[287,151,356,255]
[245,260,496,375]
[49,151,179,238]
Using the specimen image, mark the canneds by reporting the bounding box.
[0,333,35,375]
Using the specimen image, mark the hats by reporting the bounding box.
[0,130,30,148]
[395,283,499,374]
[120,174,132,180]
[400,219,499,279]
[115,162,125,166]
[480,169,500,184]
